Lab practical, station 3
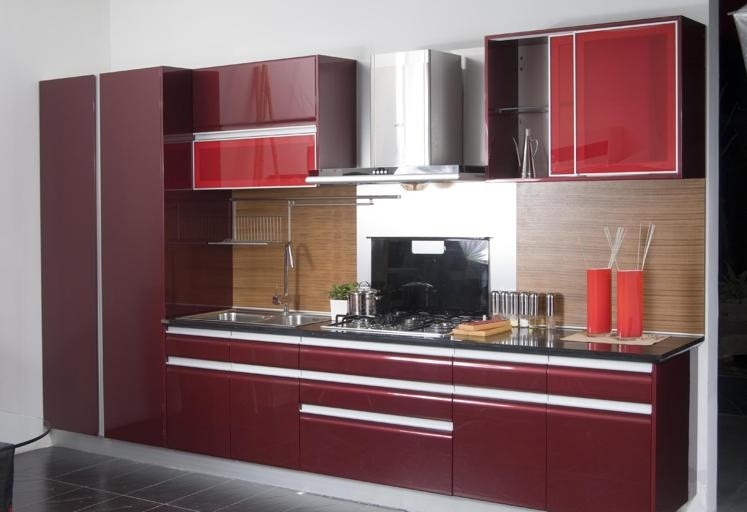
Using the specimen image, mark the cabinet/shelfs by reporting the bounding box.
[453,340,690,511]
[38,62,233,454]
[485,14,706,178]
[165,321,299,471]
[298,329,454,494]
[189,56,357,191]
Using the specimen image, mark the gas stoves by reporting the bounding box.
[320,309,482,339]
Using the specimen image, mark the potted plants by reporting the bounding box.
[325,281,360,321]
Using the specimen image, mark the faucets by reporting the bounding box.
[273,241,294,317]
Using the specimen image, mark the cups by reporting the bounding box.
[586,268,612,335]
[615,269,644,339]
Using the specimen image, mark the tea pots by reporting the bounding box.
[512,128,538,177]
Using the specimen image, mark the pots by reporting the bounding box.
[347,281,382,318]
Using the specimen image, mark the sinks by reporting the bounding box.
[189,312,274,324]
[256,315,322,327]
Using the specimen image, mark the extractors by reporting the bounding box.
[305,49,487,192]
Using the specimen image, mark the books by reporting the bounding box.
[453,319,513,337]
